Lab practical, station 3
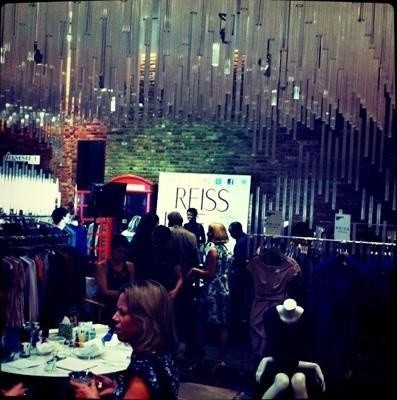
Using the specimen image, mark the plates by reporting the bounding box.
[55,358,98,371]
[6,359,40,370]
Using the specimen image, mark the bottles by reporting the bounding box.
[79,327,97,347]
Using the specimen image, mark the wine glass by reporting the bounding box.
[46,335,67,364]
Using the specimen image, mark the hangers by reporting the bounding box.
[250,233,393,258]
[3,233,68,259]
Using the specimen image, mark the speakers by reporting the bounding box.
[77,138,106,190]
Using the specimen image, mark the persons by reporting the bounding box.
[43,203,257,350]
[248,319,326,400]
[66,277,179,399]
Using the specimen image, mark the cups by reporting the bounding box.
[21,342,32,356]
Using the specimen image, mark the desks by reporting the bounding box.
[0,327,134,382]
